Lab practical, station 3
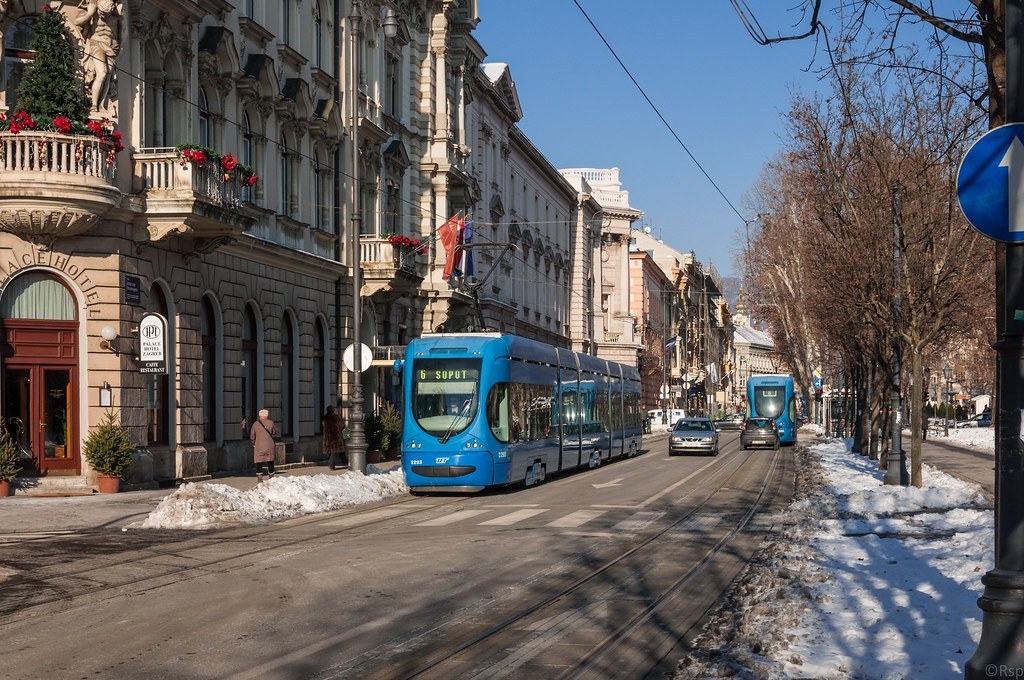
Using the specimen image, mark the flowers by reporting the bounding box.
[381,229,429,256]
[0,110,125,154]
[175,143,260,187]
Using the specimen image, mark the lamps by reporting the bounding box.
[100,326,117,349]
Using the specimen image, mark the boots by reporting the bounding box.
[267,471,275,479]
[256,472,263,483]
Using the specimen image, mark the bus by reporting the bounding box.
[744,373,798,445]
[393,330,643,494]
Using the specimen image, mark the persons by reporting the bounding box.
[442,395,462,415]
[701,422,706,429]
[322,406,345,471]
[643,415,651,433]
[512,416,521,442]
[251,410,277,484]
[74,0,120,115]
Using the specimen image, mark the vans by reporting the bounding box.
[648,408,685,431]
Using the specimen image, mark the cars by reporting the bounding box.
[666,417,722,456]
[739,417,782,450]
[957,412,993,429]
[713,413,744,431]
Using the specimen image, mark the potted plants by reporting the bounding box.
[81,394,139,494]
[0,414,24,498]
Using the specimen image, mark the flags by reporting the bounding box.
[666,337,676,352]
[706,363,718,383]
[438,215,474,280]
